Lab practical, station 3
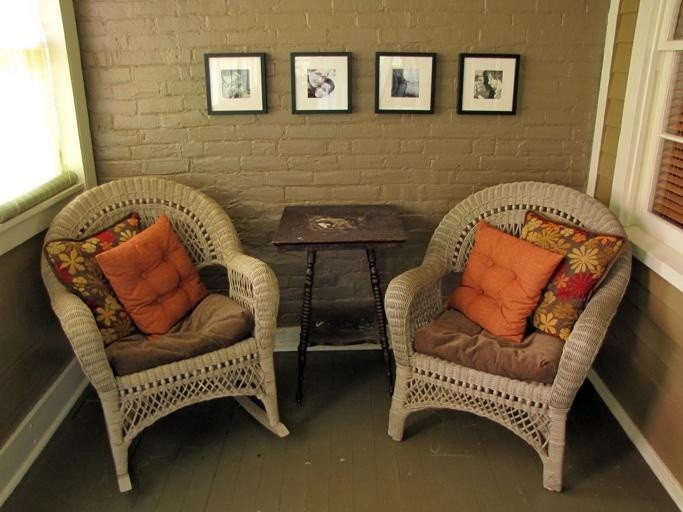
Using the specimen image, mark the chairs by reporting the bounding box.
[384,180,633,493]
[39,177,291,493]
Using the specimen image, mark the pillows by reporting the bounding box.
[43,209,212,349]
[442,207,628,346]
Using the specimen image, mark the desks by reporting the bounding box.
[271,204,408,404]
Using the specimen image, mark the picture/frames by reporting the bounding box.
[456,52,520,115]
[374,52,437,115]
[204,52,267,115]
[290,52,352,115]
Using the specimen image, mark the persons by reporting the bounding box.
[308,69,329,98]
[315,78,334,98]
